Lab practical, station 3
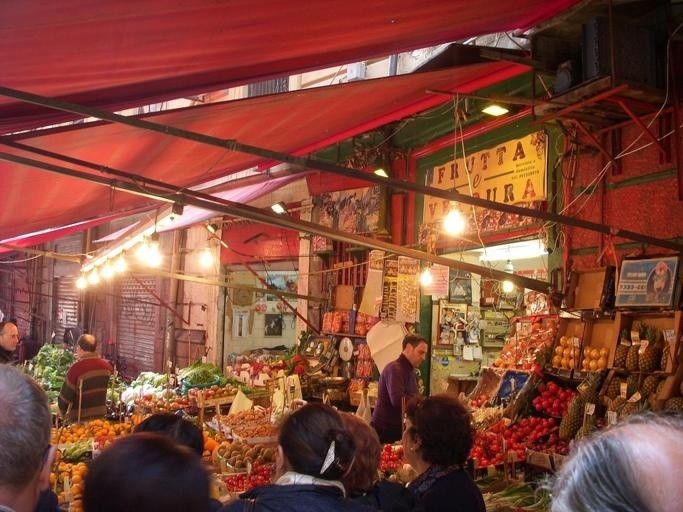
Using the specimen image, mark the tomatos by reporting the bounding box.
[224,461,272,492]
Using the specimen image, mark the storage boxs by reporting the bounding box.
[349,381,379,409]
[302,334,338,360]
[466,266,682,472]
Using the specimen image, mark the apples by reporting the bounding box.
[469,380,576,468]
[378,443,408,470]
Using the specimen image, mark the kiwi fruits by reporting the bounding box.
[218,439,277,468]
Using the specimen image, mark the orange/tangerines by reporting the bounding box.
[50,415,145,512]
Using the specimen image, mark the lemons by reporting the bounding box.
[551,335,609,374]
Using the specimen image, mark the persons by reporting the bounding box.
[0,322,18,363]
[0,361,683,511]
[371,334,429,439]
[58,334,113,423]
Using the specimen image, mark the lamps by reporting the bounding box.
[442,110,467,236]
[76,205,163,290]
[271,202,290,217]
[480,102,511,116]
[206,223,218,234]
[374,166,392,178]
[172,203,184,215]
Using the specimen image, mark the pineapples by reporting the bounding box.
[558,320,683,443]
